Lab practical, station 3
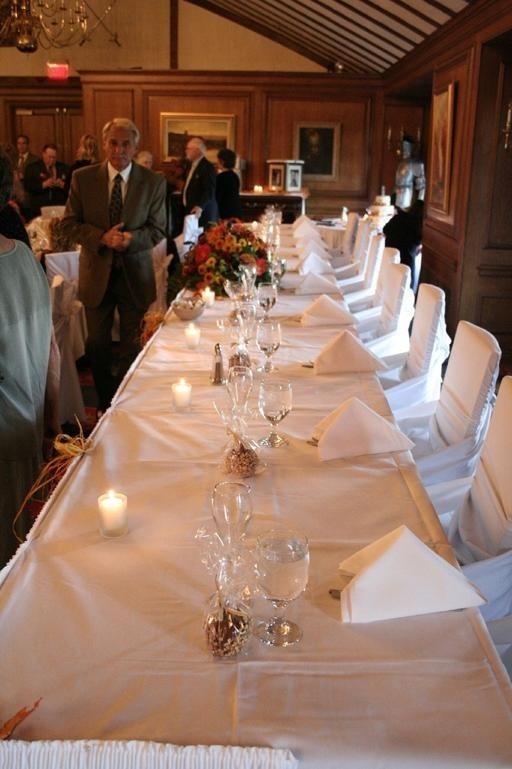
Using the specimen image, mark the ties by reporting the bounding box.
[19,156,24,168]
[109,173,122,228]
[48,165,53,178]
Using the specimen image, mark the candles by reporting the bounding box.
[199,283,218,308]
[251,220,258,229]
[254,183,265,193]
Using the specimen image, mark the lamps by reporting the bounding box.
[0,1,122,54]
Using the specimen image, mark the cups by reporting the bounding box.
[172,383,194,412]
[185,329,201,349]
[202,291,215,309]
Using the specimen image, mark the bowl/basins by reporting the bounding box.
[171,299,207,322]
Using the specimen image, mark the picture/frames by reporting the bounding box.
[285,163,303,194]
[291,119,343,181]
[268,164,285,192]
[159,112,237,168]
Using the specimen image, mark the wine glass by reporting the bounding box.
[224,258,287,382]
[255,529,309,649]
[207,482,254,610]
[257,380,292,449]
[261,205,283,249]
[29,208,59,252]
[226,367,252,415]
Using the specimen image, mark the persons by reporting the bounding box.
[0,148,32,251]
[180,138,219,232]
[213,148,239,220]
[66,132,97,192]
[60,118,167,408]
[10,134,38,179]
[0,231,54,570]
[26,142,68,208]
[137,149,153,171]
